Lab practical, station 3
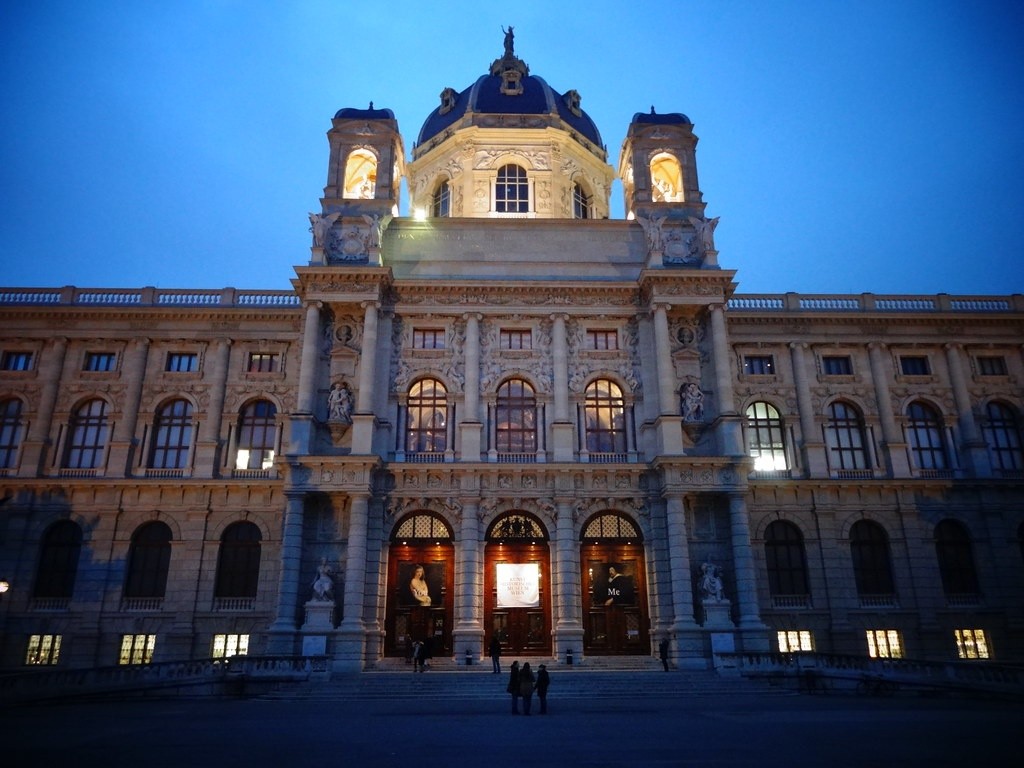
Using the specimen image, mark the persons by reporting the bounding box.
[310,556,337,600]
[699,217,713,250]
[648,216,662,250]
[507,660,550,716]
[314,213,326,246]
[701,555,724,601]
[659,637,669,672]
[371,215,381,247]
[488,637,501,674]
[685,385,705,421]
[328,384,351,421]
[404,634,425,673]
